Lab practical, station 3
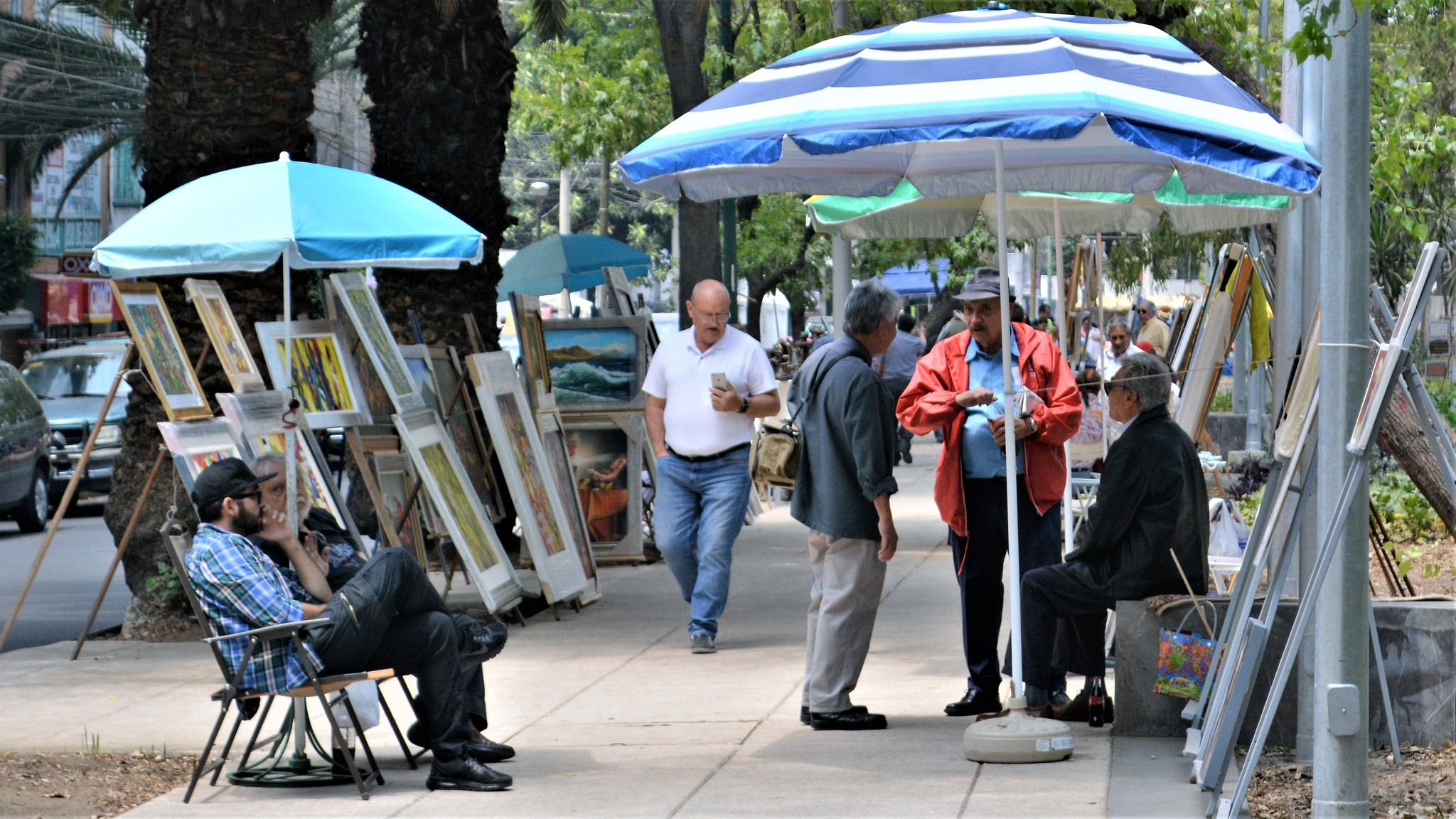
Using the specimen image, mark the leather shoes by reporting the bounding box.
[975,705,1053,723]
[942,686,1002,715]
[1048,687,1072,707]
[1050,689,1114,723]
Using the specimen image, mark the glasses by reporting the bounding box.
[1103,379,1128,395]
[691,305,730,324]
[1136,308,1146,315]
[1112,335,1124,340]
[230,490,262,504]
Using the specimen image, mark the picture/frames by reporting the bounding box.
[1389,237,1441,345]
[1275,302,1319,460]
[108,270,645,626]
[1344,343,1402,455]
[1193,616,1268,787]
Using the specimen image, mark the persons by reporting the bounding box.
[895,266,1211,723]
[186,456,517,794]
[640,278,780,653]
[785,277,926,732]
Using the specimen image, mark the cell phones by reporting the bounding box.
[711,372,727,393]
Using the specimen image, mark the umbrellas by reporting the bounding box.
[610,7,1324,697]
[87,151,486,758]
[871,256,952,309]
[496,231,650,317]
[802,180,1299,370]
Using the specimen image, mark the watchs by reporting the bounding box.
[737,397,748,413]
[1031,417,1038,435]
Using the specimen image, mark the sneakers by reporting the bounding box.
[426,750,513,792]
[457,619,507,672]
[690,631,717,655]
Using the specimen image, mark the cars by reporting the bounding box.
[0,341,130,532]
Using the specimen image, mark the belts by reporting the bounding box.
[664,441,752,463]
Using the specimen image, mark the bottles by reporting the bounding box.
[987,399,1019,459]
[331,701,355,777]
[1088,676,1105,727]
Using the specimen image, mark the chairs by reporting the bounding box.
[159,518,430,803]
[1073,469,1102,546]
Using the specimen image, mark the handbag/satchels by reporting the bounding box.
[745,417,802,490]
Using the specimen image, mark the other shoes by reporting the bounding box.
[801,705,868,725]
[809,708,887,730]
[894,443,913,465]
[407,719,516,761]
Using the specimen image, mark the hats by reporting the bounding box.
[191,457,278,507]
[952,266,1015,302]
[1136,341,1153,352]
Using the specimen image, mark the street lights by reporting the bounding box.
[530,181,549,242]
